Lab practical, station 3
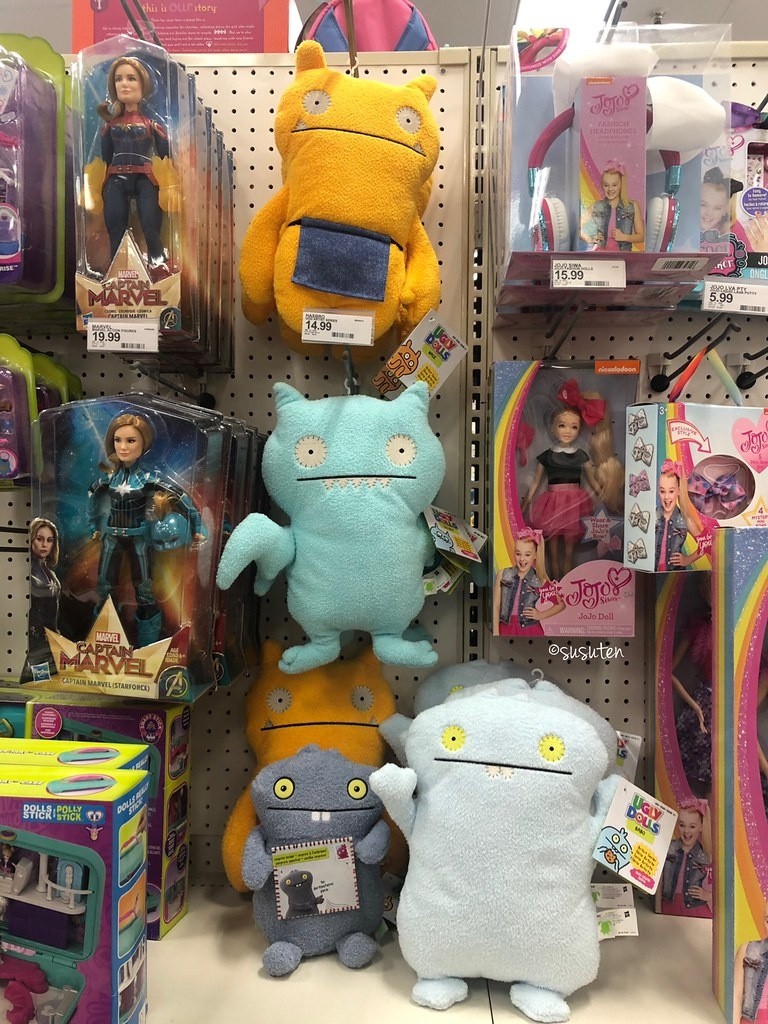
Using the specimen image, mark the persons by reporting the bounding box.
[653,459,706,571]
[699,168,744,234]
[85,415,206,648]
[655,797,713,918]
[521,378,624,582]
[579,160,644,253]
[732,902,768,1024]
[670,571,713,799]
[492,526,565,636]
[28,518,61,649]
[95,55,174,282]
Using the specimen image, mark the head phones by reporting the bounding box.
[527,106,682,249]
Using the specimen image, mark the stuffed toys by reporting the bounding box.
[219,382,447,670]
[232,40,444,361]
[221,643,400,896]
[244,743,389,974]
[375,661,627,1024]
